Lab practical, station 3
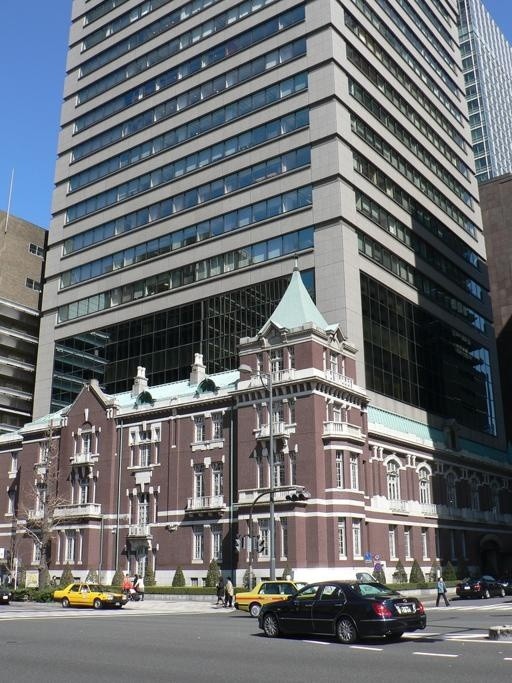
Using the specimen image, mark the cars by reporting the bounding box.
[236,578,331,617]
[257,579,427,644]
[54,582,129,608]
[455,575,512,599]
[0,588,13,605]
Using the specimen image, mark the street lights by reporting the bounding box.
[237,356,284,581]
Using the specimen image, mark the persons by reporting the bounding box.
[216,576,224,605]
[435,577,450,606]
[5,572,12,588]
[121,573,145,601]
[11,573,19,586]
[224,575,234,607]
[50,575,57,602]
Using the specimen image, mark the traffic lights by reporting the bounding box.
[285,492,309,502]
[232,538,240,552]
[258,539,265,552]
